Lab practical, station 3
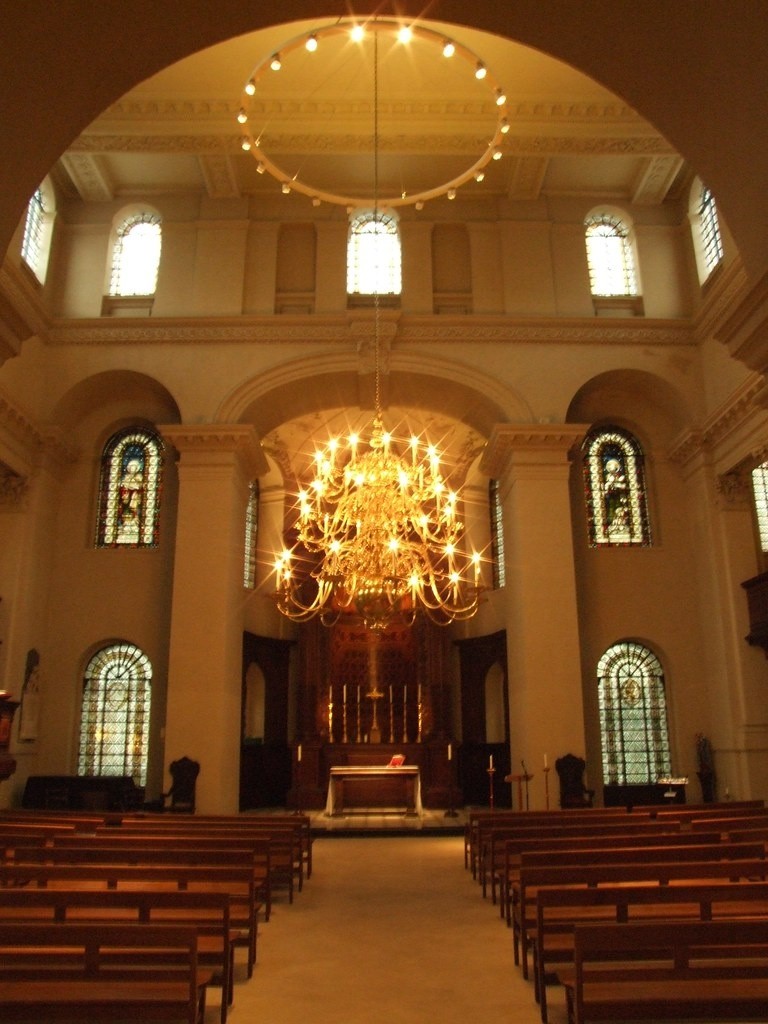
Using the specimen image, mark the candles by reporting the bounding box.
[294,684,548,772]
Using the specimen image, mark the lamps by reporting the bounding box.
[235,20,511,214]
[248,1,494,635]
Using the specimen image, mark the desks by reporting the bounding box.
[324,764,423,816]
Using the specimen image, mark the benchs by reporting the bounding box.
[0,809,313,1024]
[464,802,768,1024]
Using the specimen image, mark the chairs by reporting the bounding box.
[143,756,200,813]
[556,754,595,809]
